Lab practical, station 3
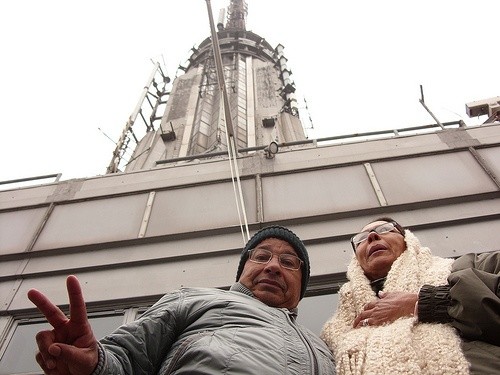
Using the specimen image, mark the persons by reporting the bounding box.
[27,225,338,375]
[322,217,500,375]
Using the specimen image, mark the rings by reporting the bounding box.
[364,320,369,327]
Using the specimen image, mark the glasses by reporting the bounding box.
[351,221,405,253]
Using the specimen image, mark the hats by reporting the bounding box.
[236,225,310,301]
[248,249,304,271]
